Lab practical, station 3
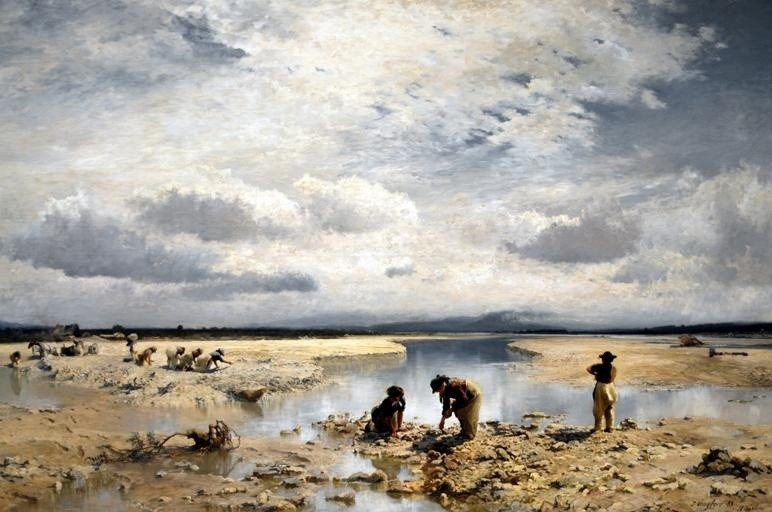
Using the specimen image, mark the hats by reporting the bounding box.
[599,352,616,358]
[431,377,445,394]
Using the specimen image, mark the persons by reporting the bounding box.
[174,348,202,372]
[429,374,483,440]
[9,369,21,396]
[125,334,138,356]
[135,346,156,367]
[8,351,23,366]
[166,345,186,367]
[74,338,85,358]
[374,459,407,501]
[370,385,407,440]
[196,347,233,372]
[586,351,618,432]
